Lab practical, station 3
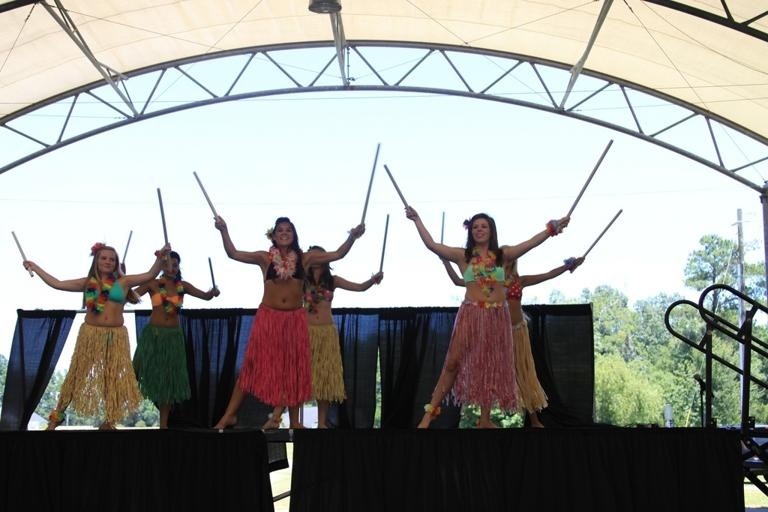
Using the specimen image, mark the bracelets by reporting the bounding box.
[545,219,562,236]
[563,257,578,274]
[370,274,380,285]
[346,230,357,243]
[154,250,168,262]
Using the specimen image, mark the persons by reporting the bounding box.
[119,250,221,429]
[261,245,385,429]
[213,215,365,429]
[23,242,172,432]
[436,253,586,429]
[402,203,572,430]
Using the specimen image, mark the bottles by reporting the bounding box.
[664,403,675,428]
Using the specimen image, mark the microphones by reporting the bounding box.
[695,372,715,399]
